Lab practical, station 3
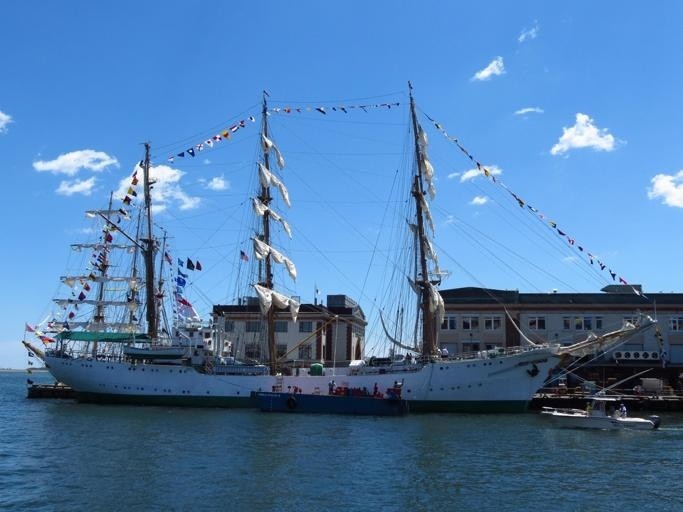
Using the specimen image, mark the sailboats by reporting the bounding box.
[20,74,657,416]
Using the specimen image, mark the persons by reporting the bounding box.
[328,380,336,395]
[392,378,404,388]
[442,345,448,355]
[312,385,320,395]
[619,404,627,418]
[373,382,379,395]
[587,402,593,412]
[406,353,412,360]
[293,384,302,394]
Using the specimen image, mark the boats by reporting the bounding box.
[247,388,411,418]
[539,392,660,430]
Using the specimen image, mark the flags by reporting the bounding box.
[35,159,143,343]
[165,251,202,321]
[433,121,640,296]
[27,323,35,385]
[168,116,255,164]
[273,102,399,115]
[240,250,249,262]
